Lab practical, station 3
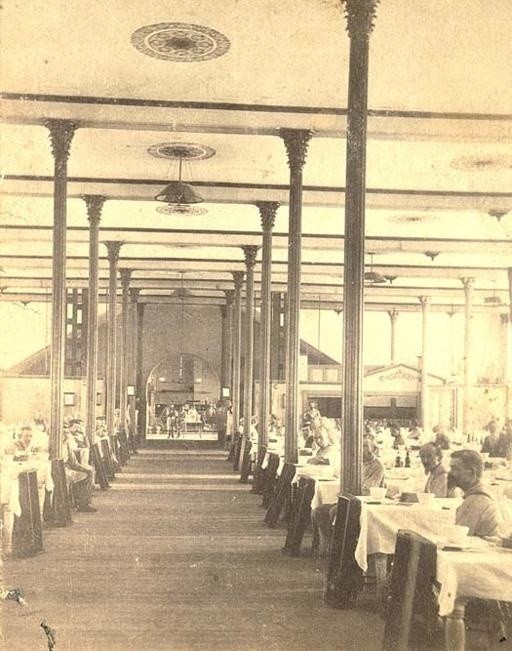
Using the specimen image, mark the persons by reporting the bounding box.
[224,397,234,448]
[248,397,511,551]
[164,400,214,440]
[0,407,131,554]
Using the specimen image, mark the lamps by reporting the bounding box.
[359,252,387,285]
[156,149,208,210]
[172,272,198,301]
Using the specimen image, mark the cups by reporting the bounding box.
[368,486,471,543]
